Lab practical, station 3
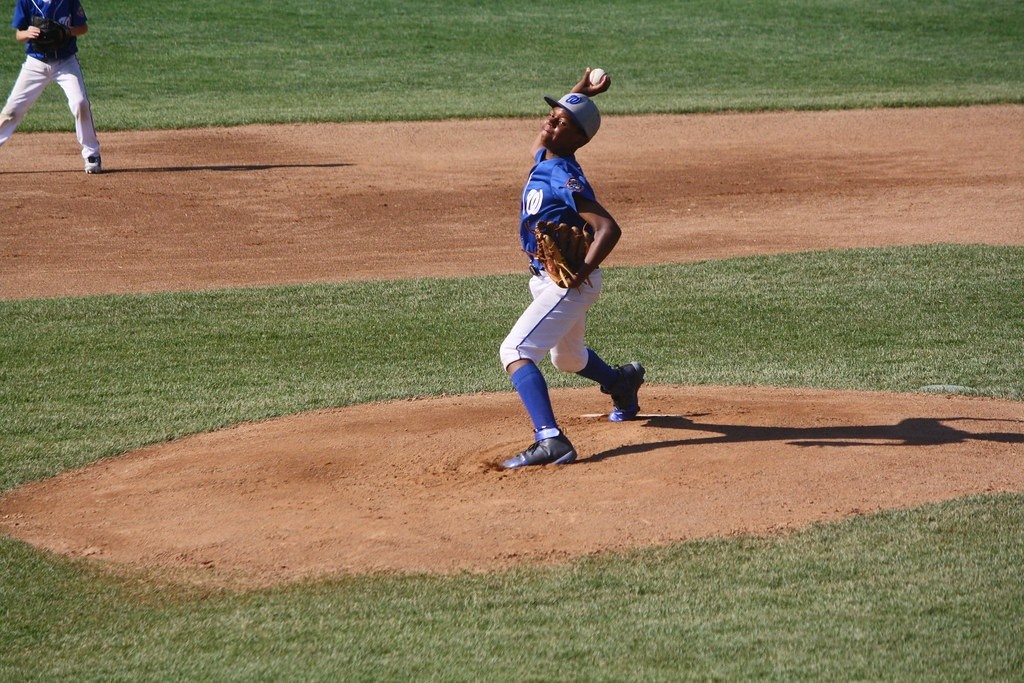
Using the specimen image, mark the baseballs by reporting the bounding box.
[589,68,608,86]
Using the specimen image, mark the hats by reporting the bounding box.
[544,93,601,139]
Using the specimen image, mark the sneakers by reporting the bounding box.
[501,428,578,469]
[84,153,102,174]
[600,361,646,421]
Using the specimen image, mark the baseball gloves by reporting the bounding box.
[518,218,595,295]
[29,16,72,51]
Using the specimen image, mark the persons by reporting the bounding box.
[0,0,101,174]
[499,66,645,471]
